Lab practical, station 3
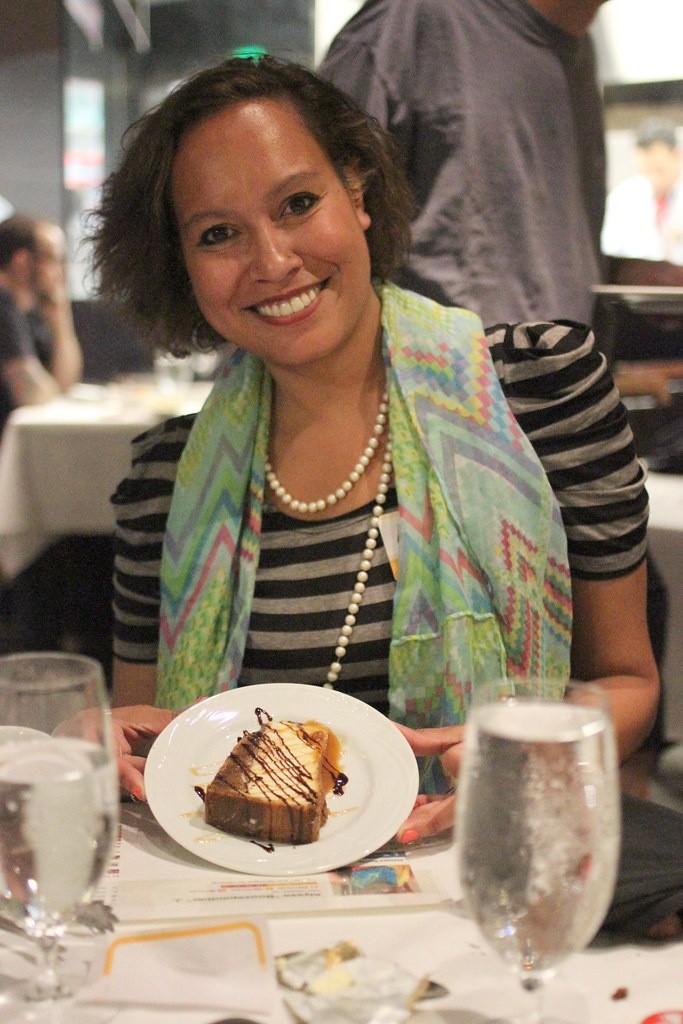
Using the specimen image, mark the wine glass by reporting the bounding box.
[0,651,122,1024]
[452,676,622,1024]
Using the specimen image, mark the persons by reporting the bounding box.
[51,52,661,841]
[0,215,82,410]
[318,0,610,342]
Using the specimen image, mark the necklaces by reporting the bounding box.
[264,391,395,693]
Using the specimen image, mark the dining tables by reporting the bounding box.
[0,371,215,586]
[0,795,683,1024]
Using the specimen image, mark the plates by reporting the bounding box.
[143,683,419,877]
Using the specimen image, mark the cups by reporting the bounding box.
[153,361,194,414]
